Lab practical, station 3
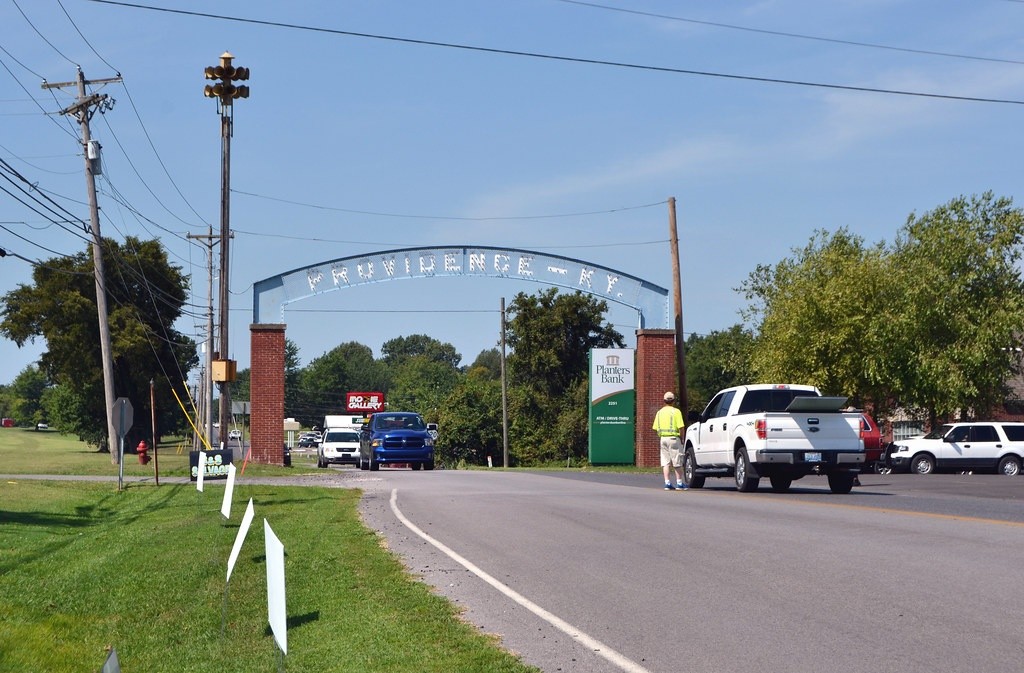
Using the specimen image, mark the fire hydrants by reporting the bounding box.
[137,440,152,465]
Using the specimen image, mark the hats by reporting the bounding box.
[664,391,675,400]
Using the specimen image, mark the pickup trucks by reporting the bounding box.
[684,383,867,495]
[359,411,437,471]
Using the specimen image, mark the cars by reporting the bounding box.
[212,422,219,428]
[297,431,322,448]
[859,412,893,475]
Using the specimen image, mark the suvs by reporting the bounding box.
[317,428,361,468]
[885,421,1024,475]
[228,429,242,441]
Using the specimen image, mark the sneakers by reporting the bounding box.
[664,483,675,490]
[676,482,689,491]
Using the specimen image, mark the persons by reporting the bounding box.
[653,392,689,491]
